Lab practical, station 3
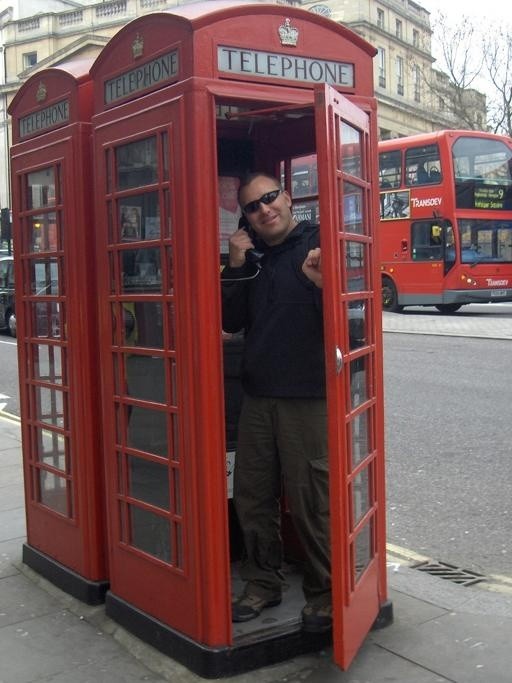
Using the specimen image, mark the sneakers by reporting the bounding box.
[300,602,333,635]
[232,591,282,621]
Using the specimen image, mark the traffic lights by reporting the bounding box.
[1,206,11,239]
[42,186,49,204]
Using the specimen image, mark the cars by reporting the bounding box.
[0,256,58,337]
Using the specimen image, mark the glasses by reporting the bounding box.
[239,188,280,216]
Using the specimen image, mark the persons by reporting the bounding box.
[218,167,334,631]
[121,208,141,240]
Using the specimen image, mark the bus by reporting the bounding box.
[279,130,511,313]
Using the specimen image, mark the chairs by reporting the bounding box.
[381,166,441,187]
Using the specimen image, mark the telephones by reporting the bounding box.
[238,220,265,264]
